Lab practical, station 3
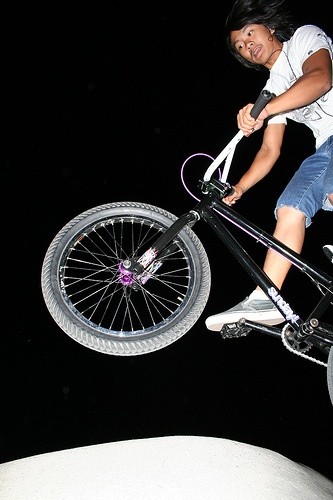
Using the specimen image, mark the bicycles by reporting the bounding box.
[40,89,333,410]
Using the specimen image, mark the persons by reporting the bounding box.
[205,0,333,331]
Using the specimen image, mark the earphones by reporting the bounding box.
[268,28,275,35]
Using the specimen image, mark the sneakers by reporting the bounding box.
[205,296,286,331]
[322,244,333,263]
